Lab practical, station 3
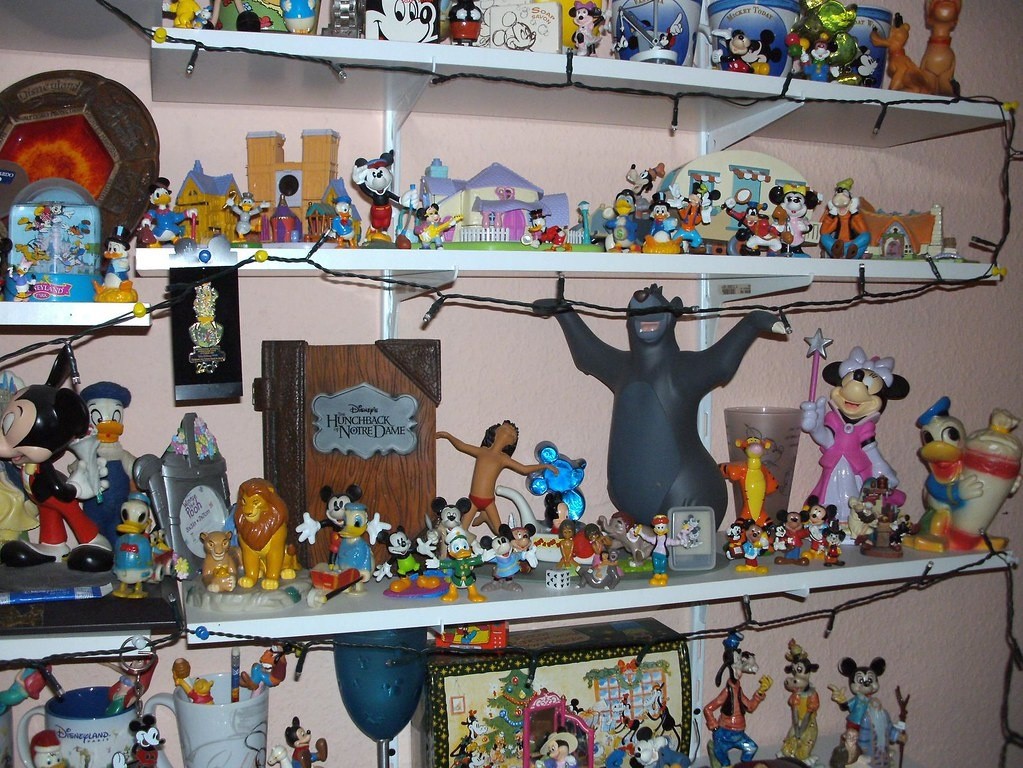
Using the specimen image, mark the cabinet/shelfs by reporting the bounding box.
[0,297,151,666]
[148,23,1017,647]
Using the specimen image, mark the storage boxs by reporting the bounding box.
[425,616,695,768]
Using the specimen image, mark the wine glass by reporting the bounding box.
[334,627,427,767]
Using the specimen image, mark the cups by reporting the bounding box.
[724,406,803,527]
[143,672,269,768]
[16,687,136,768]
